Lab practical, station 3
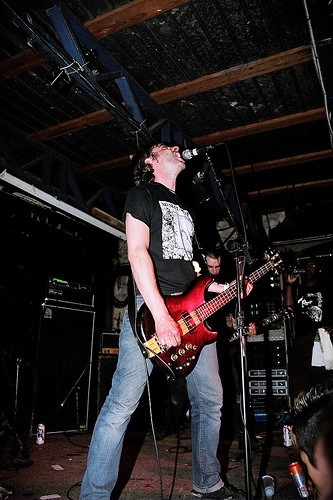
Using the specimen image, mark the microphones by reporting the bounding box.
[182,144,219,160]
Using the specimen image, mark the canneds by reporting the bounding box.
[283,425,293,447]
[289,462,311,498]
[36,424,45,445]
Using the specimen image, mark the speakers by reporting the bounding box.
[8,301,96,438]
[90,354,118,425]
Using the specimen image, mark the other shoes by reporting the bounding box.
[192,486,244,500]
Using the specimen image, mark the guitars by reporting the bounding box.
[228,291,296,343]
[138,247,285,379]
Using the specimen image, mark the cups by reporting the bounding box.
[262,475,274,496]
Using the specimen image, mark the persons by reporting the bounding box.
[203,246,333,500]
[78,141,255,500]
